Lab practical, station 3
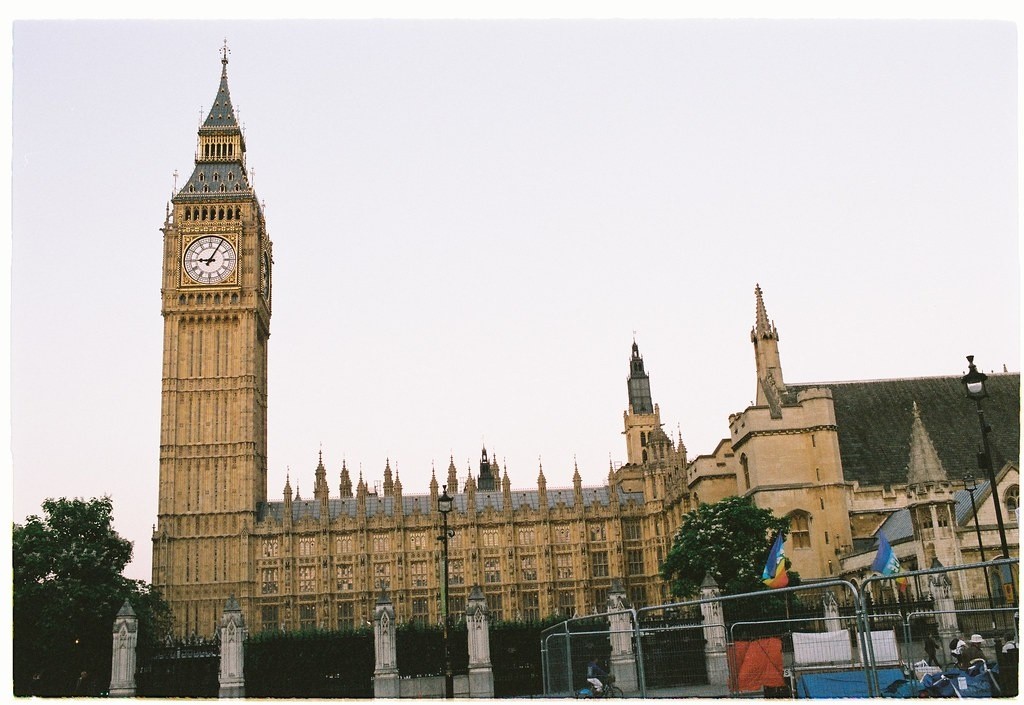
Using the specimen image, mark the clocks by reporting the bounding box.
[262,249,271,304]
[185,236,236,284]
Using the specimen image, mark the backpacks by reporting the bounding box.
[949,638,960,650]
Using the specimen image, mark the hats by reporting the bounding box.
[969,634,984,642]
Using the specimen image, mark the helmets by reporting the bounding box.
[578,689,593,698]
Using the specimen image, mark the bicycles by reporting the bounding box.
[575,674,624,697]
[944,661,961,673]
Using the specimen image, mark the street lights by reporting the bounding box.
[434,484,456,698]
[959,356,1011,557]
[962,470,998,627]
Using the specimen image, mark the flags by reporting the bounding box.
[758,530,788,589]
[869,529,907,594]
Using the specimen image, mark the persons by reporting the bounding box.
[925,632,1019,671]
[587,656,611,696]
